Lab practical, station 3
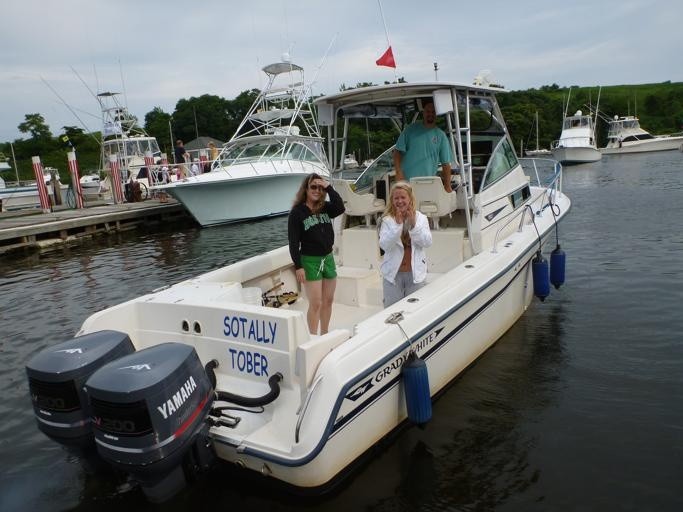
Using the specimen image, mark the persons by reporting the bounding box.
[287,173,345,335]
[378,180,432,309]
[390,102,452,228]
[205,141,219,171]
[175,140,191,179]
[157,160,173,203]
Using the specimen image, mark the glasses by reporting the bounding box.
[310,185,323,189]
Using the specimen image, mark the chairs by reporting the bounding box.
[331,178,385,228]
[410,176,457,229]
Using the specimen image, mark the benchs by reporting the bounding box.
[424,272,444,286]
[300,264,376,307]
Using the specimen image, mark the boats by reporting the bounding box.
[39,166,60,186]
[145,59,331,232]
[1,143,71,212]
[77,58,181,202]
[551,84,605,164]
[583,92,683,155]
[341,152,360,169]
[23,58,571,489]
[361,158,377,168]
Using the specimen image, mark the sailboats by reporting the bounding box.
[524,111,554,156]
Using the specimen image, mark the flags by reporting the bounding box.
[376,46,397,68]
